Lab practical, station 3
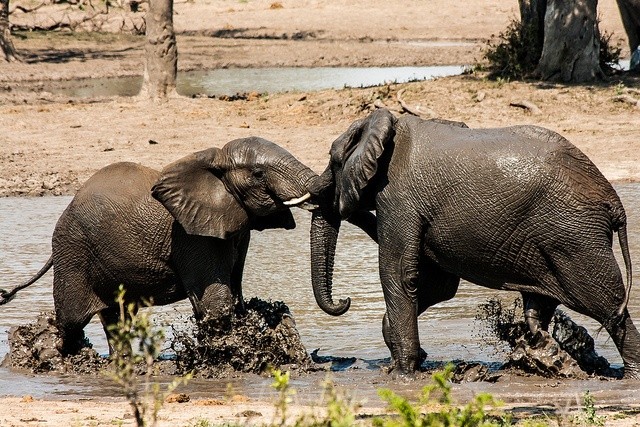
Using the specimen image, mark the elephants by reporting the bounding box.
[283,108,640,379]
[0,136,379,360]
